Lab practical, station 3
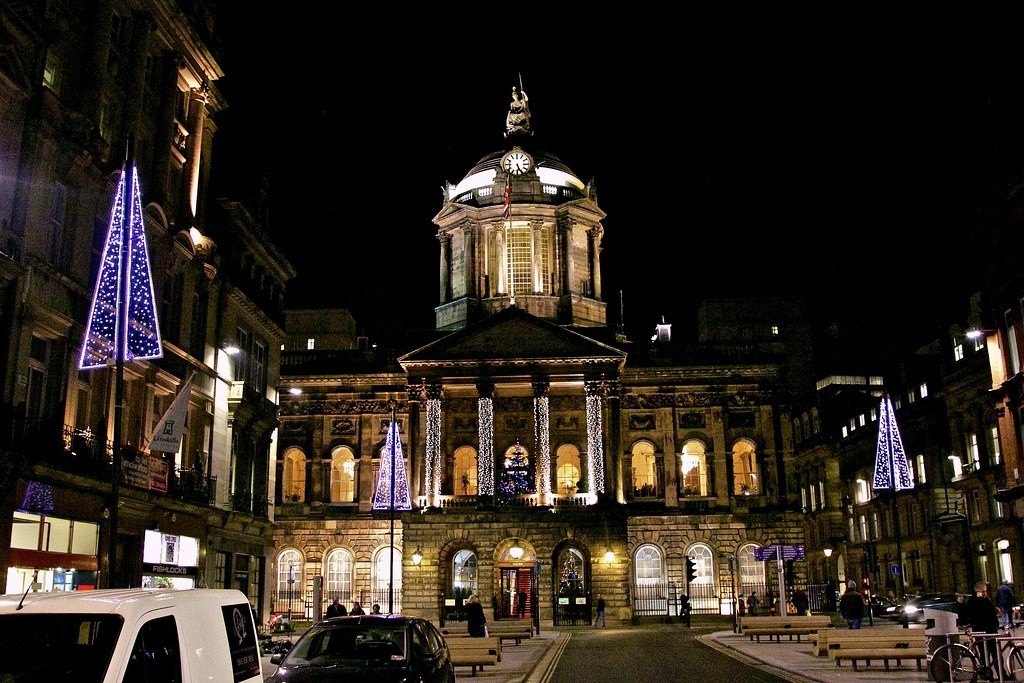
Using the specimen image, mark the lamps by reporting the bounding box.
[604,544,615,567]
[823,542,834,557]
[411,544,423,567]
[510,538,523,558]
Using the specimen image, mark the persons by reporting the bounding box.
[967,582,1016,681]
[370,604,382,615]
[517,589,527,619]
[769,591,778,615]
[506,92,531,129]
[791,587,809,616]
[953,592,967,628]
[326,596,348,617]
[679,590,687,628]
[840,580,866,629]
[738,593,746,617]
[740,482,749,495]
[996,579,1018,628]
[465,596,487,677]
[349,602,365,616]
[685,484,700,495]
[747,591,761,616]
[593,595,606,628]
[634,483,656,497]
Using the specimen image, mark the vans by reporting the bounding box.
[0,588,263,683]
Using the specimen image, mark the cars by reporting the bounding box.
[264,616,456,683]
[872,593,971,624]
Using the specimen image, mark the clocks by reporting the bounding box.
[504,151,529,176]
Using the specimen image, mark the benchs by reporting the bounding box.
[735,616,926,670]
[441,620,533,675]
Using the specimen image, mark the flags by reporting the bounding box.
[503,172,510,219]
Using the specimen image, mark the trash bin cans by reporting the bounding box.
[918,601,961,682]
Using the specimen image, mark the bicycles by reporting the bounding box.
[931,624,1024,683]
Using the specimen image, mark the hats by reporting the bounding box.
[848,580,856,588]
